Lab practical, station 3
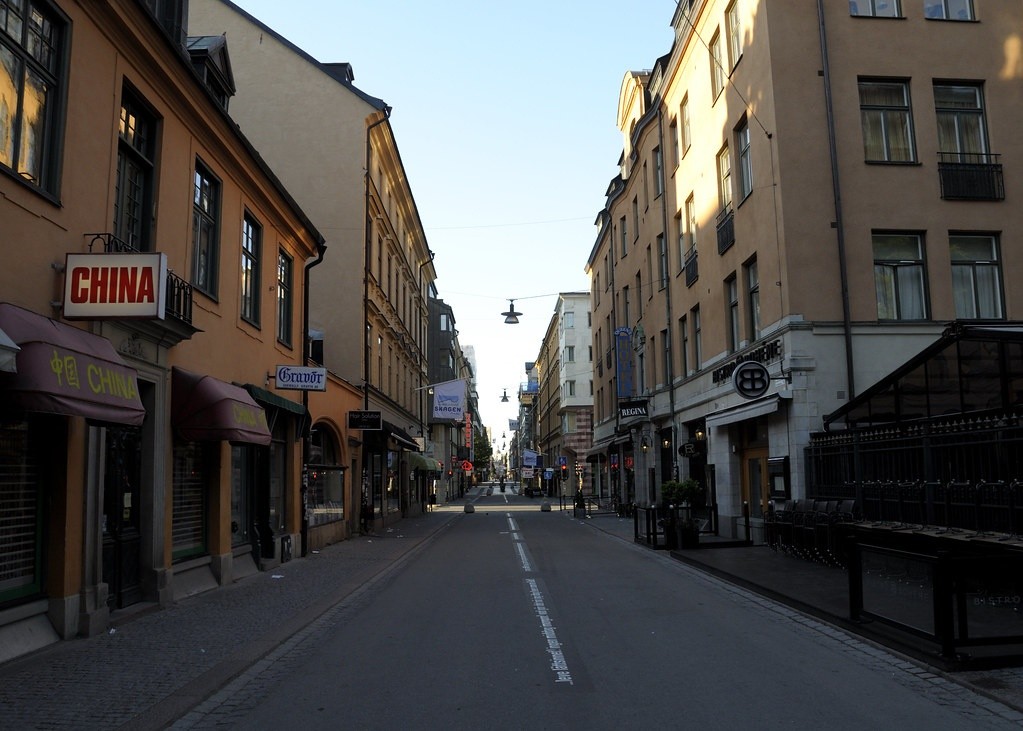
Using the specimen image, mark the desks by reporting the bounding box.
[852,521,1023,554]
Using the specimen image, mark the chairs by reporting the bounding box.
[764,498,865,569]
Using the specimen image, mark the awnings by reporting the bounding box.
[379,419,420,453]
[410,452,441,480]
[172,365,306,447]
[589,440,615,455]
[0,302,146,426]
[586,453,607,463]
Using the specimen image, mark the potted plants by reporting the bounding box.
[658,480,702,550]
[573,489,585,518]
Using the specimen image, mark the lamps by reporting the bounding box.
[501,298,523,323]
[662,437,672,448]
[499,388,510,402]
[696,423,710,440]
[641,435,653,454]
